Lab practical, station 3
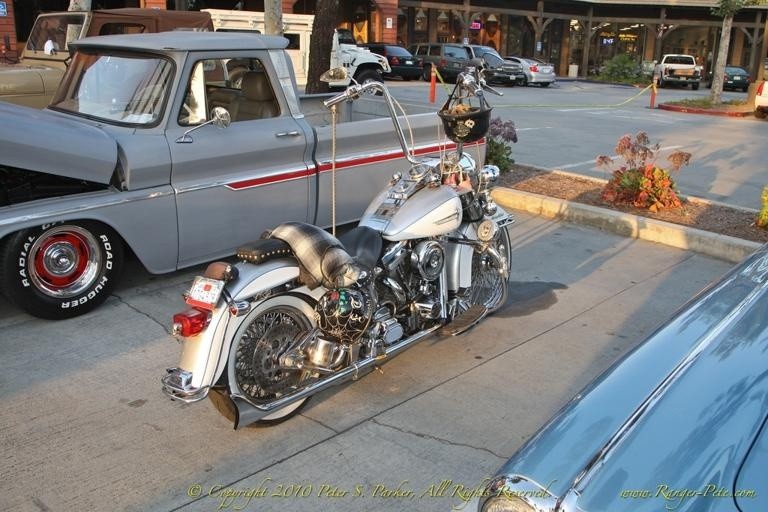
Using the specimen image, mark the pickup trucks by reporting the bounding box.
[1,30,489,325]
[654,52,704,90]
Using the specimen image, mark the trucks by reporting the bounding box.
[198,8,392,95]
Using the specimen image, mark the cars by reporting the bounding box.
[502,55,557,89]
[460,44,524,86]
[639,59,655,79]
[755,79,768,119]
[705,65,751,93]
[446,236,767,512]
[364,42,420,81]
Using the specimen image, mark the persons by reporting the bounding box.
[476,14,500,54]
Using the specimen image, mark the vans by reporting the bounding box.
[0,8,229,110]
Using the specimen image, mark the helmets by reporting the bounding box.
[438,107,490,143]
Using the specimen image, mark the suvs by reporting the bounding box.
[406,42,471,81]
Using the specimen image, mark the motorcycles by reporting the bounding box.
[160,63,513,433]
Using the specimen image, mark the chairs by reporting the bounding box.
[229,72,279,124]
